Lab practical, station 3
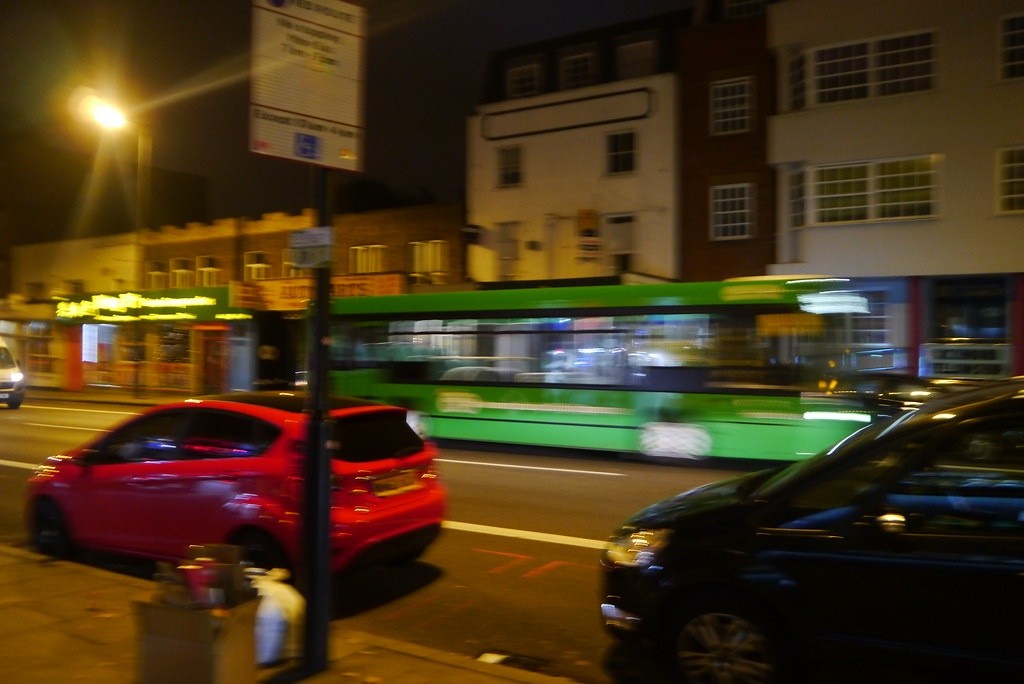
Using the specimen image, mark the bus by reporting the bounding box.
[301,275,905,462]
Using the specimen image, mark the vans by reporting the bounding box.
[0,337,23,409]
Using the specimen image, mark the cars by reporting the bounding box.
[600,378,1024,681]
[20,386,448,602]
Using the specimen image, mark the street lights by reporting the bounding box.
[86,95,149,389]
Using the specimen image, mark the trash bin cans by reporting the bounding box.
[126,586,264,684]
